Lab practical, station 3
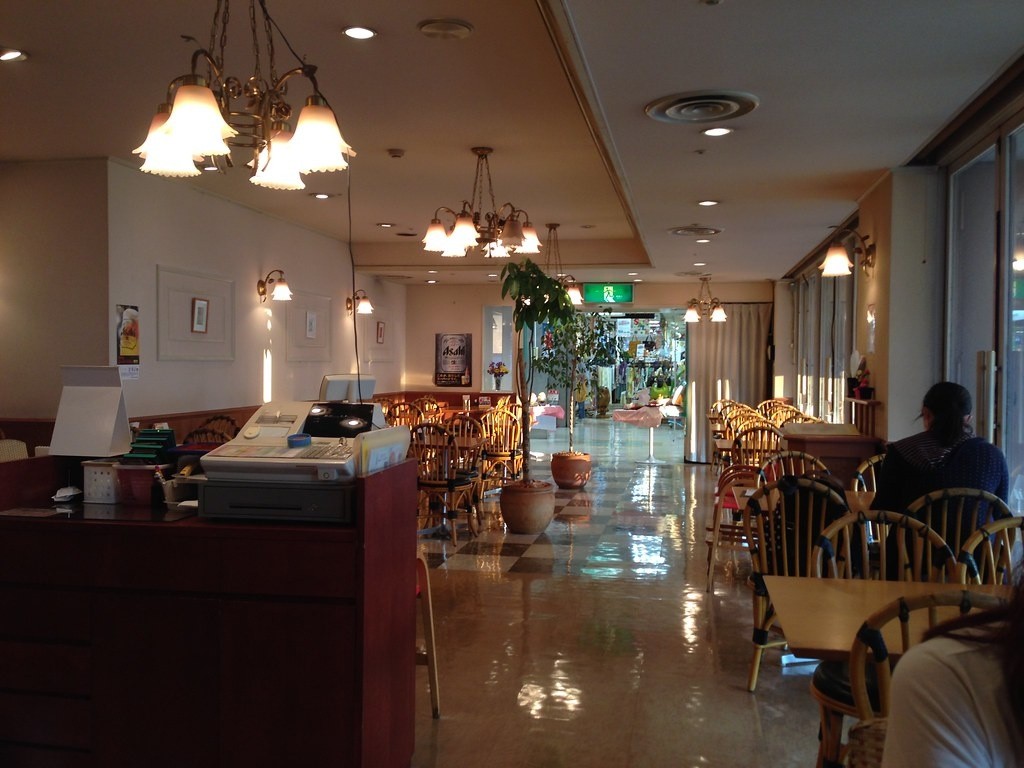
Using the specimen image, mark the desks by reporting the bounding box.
[730,485,878,520]
[440,406,489,413]
[411,435,489,467]
[716,438,780,450]
[613,406,677,465]
[529,406,564,459]
[761,573,1013,658]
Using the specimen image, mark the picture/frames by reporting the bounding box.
[192,297,210,333]
[376,321,385,343]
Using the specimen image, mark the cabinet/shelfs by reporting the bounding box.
[0,454,419,768]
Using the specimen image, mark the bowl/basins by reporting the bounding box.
[113,463,170,505]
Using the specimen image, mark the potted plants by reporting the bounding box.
[497,260,617,537]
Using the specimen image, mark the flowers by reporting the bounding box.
[487,361,509,390]
[857,369,870,387]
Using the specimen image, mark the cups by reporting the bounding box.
[417,426,427,440]
[462,395,471,411]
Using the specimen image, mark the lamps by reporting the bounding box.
[684,277,726,323]
[132,0,584,316]
[818,226,873,277]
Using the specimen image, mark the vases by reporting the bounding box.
[496,378,502,390]
[853,386,873,399]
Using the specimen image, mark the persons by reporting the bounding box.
[576,374,588,419]
[881,555,1024,768]
[577,357,590,388]
[853,357,869,399]
[150,465,166,507]
[869,381,1008,585]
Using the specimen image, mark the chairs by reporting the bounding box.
[373,394,524,720]
[182,414,240,450]
[705,397,1024,768]
